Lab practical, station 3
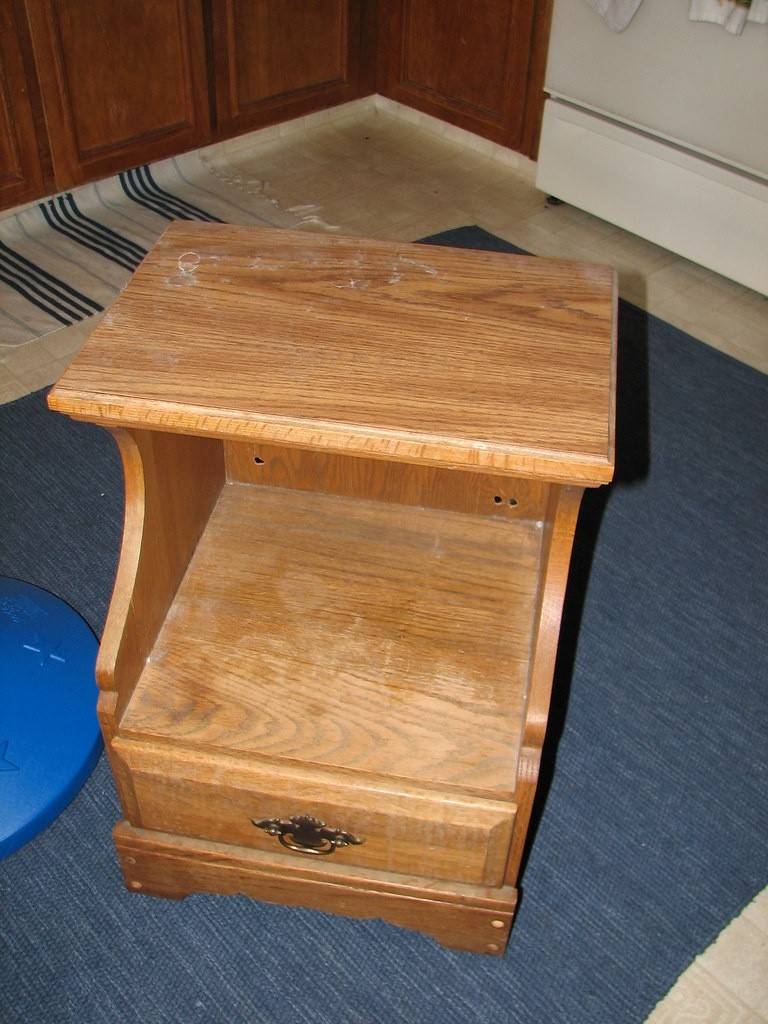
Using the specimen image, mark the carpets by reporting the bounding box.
[0,143,338,347]
[0,221,768,1024]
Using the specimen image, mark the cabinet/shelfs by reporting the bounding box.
[0,0,555,210]
[48,220,621,958]
